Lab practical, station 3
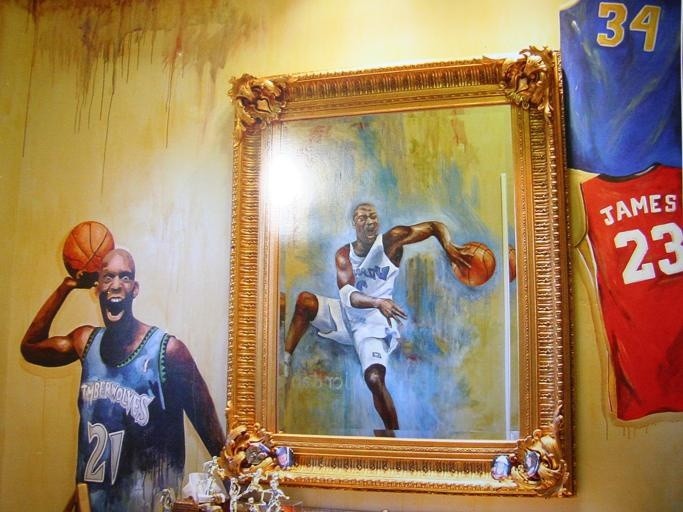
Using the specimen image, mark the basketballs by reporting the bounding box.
[63,222,115,279]
[454,242,496,286]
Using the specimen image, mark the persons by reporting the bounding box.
[19,248,225,512]
[280,200,473,438]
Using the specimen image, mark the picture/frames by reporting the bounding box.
[210,42,579,499]
